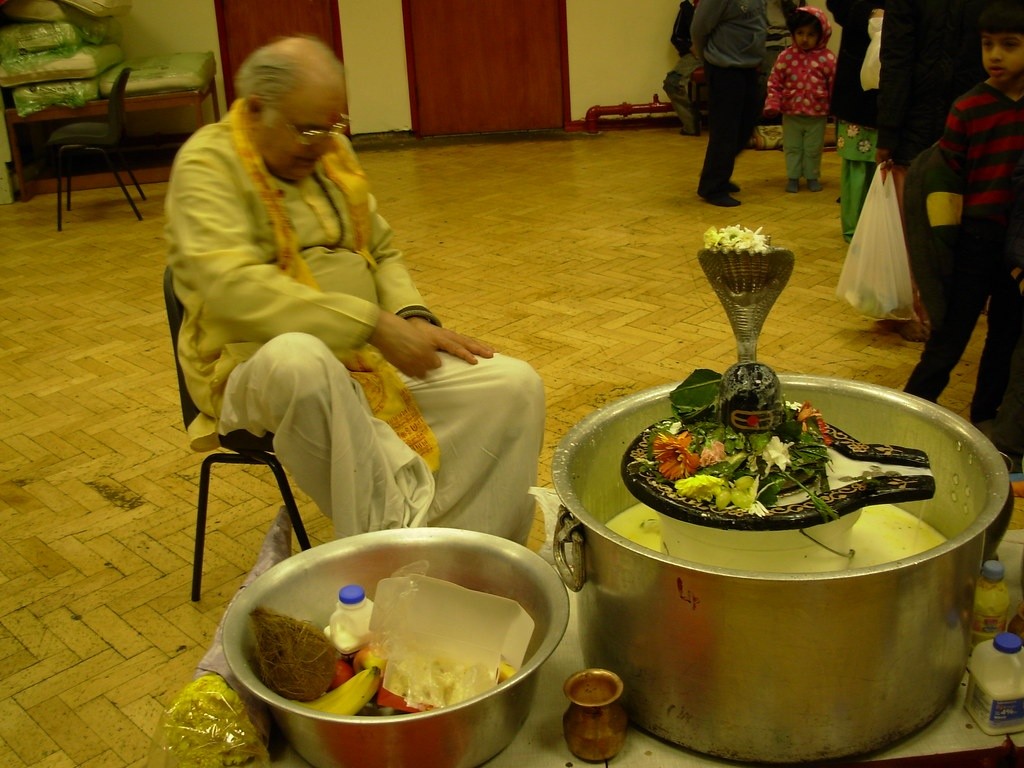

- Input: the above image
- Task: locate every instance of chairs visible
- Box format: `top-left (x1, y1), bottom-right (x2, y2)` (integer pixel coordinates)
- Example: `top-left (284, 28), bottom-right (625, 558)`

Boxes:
top-left (161, 262), bottom-right (311, 600)
top-left (45, 66), bottom-right (146, 232)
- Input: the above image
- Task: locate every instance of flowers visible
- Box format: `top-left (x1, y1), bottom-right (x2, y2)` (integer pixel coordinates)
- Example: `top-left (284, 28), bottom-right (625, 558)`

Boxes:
top-left (627, 368), bottom-right (843, 524)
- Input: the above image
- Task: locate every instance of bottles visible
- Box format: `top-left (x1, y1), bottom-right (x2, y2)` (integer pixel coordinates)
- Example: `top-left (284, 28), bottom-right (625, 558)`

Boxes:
top-left (968, 561), bottom-right (1010, 656)
top-left (964, 634), bottom-right (1024, 735)
top-left (323, 585), bottom-right (377, 665)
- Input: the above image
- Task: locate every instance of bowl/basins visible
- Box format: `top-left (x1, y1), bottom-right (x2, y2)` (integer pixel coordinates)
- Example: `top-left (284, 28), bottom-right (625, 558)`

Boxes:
top-left (224, 526), bottom-right (572, 767)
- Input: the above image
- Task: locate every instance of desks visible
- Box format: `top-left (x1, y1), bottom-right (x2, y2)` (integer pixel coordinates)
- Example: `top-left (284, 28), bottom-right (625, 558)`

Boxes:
top-left (482, 529), bottom-right (1024, 768)
top-left (5, 78), bottom-right (222, 201)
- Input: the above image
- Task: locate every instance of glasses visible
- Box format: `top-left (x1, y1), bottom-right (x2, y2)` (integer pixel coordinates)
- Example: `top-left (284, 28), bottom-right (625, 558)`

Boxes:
top-left (266, 103), bottom-right (350, 145)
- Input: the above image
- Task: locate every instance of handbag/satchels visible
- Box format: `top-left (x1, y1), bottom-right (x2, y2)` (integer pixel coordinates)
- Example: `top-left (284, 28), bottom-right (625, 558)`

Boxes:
top-left (837, 162), bottom-right (914, 321)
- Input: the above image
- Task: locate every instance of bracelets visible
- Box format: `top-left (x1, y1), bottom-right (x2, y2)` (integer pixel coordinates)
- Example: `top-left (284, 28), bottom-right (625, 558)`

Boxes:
top-left (399, 307), bottom-right (442, 328)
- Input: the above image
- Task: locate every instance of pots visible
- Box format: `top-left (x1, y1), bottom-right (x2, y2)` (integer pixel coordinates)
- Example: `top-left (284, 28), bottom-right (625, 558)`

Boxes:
top-left (552, 369), bottom-right (1015, 762)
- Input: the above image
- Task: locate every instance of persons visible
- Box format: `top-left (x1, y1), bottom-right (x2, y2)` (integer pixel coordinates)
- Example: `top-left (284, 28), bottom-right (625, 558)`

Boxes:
top-left (164, 35), bottom-right (545, 548)
top-left (827, 0), bottom-right (1024, 474)
top-left (662, 0), bottom-right (700, 137)
top-left (763, 5), bottom-right (838, 193)
top-left (688, 0), bottom-right (768, 207)
